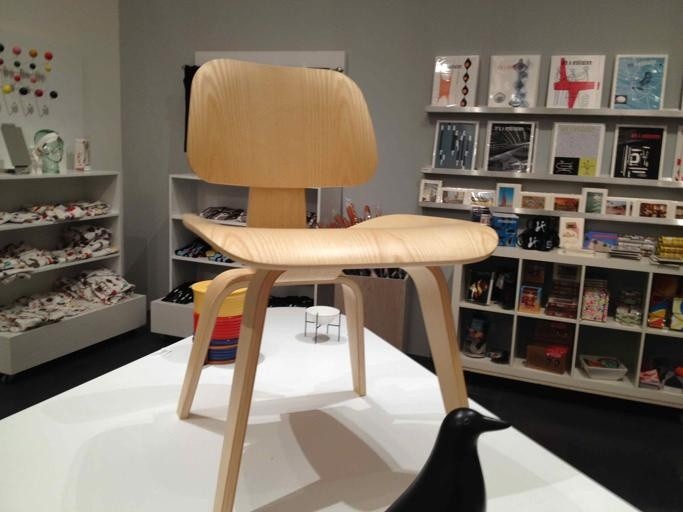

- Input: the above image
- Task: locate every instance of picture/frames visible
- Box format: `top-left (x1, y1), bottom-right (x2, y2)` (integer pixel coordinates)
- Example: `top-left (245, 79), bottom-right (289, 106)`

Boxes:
top-left (416, 118), bottom-right (683, 224)
top-left (608, 53), bottom-right (670, 112)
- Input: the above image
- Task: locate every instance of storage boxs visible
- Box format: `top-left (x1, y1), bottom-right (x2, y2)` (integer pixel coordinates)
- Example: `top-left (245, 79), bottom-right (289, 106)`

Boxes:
top-left (525, 340), bottom-right (569, 375)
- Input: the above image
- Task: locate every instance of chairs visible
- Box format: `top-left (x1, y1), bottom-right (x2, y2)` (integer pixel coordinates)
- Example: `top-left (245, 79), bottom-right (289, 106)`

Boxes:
top-left (176, 55), bottom-right (499, 512)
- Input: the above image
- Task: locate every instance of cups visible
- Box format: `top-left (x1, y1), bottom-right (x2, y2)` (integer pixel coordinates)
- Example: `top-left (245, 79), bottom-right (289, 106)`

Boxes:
top-left (72, 137), bottom-right (89, 170)
top-left (190, 279), bottom-right (249, 365)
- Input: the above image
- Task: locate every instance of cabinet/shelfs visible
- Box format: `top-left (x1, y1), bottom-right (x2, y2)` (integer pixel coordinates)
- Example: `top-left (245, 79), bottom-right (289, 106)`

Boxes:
top-left (416, 98), bottom-right (683, 229)
top-left (146, 172), bottom-right (346, 341)
top-left (450, 242), bottom-right (681, 413)
top-left (1, 166), bottom-right (146, 377)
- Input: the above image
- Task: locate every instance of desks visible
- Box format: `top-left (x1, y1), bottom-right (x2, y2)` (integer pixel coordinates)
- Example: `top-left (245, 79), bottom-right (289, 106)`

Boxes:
top-left (0, 302), bottom-right (643, 512)
